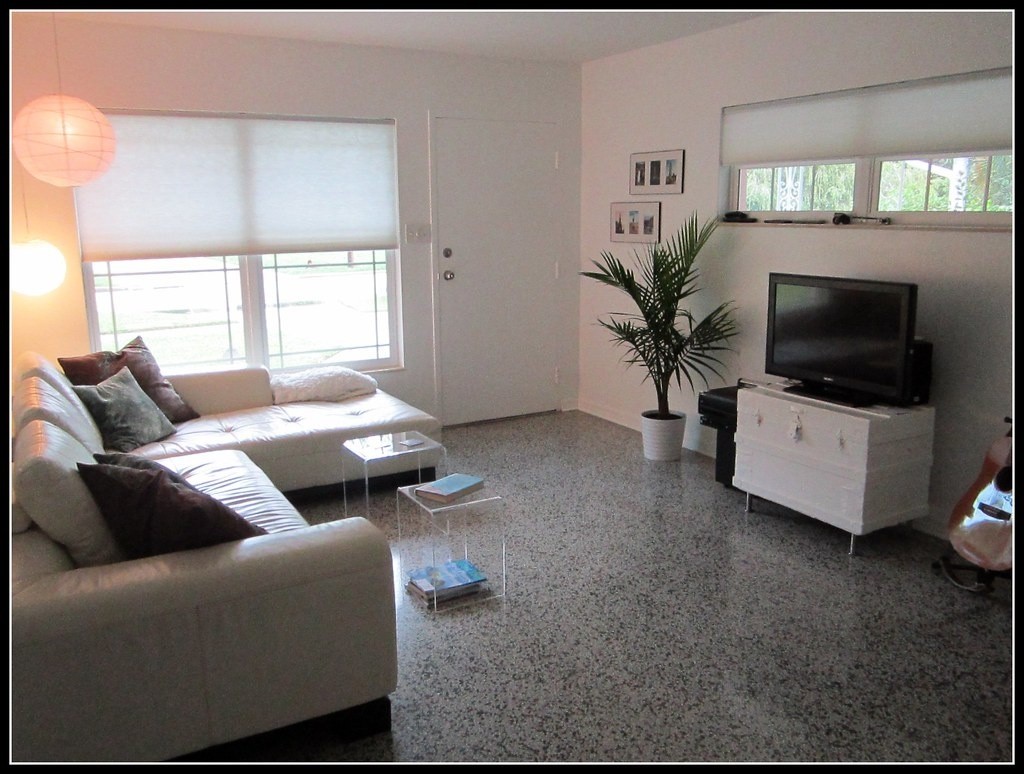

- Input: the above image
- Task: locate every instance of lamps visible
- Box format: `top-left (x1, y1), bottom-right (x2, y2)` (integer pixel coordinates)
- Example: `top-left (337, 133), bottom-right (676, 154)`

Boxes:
top-left (13, 12), bottom-right (116, 188)
top-left (11, 167), bottom-right (68, 297)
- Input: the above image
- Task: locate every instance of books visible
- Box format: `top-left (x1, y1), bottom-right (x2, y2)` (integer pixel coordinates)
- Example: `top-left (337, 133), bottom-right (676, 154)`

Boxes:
top-left (400, 558), bottom-right (487, 610)
top-left (415, 472), bottom-right (486, 506)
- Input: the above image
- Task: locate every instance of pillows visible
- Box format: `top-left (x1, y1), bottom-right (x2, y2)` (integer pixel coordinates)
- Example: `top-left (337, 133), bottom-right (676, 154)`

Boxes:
top-left (57, 336), bottom-right (201, 424)
top-left (75, 461), bottom-right (270, 559)
top-left (71, 365), bottom-right (177, 453)
top-left (92, 453), bottom-right (201, 492)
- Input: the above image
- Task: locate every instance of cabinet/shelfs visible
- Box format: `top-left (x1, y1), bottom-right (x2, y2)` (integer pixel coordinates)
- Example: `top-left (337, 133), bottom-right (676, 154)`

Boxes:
top-left (731, 382), bottom-right (936, 556)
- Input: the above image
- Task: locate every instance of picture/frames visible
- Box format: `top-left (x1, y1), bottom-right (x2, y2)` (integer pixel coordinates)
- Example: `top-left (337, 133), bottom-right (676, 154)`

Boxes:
top-left (609, 201), bottom-right (661, 244)
top-left (628, 149), bottom-right (685, 195)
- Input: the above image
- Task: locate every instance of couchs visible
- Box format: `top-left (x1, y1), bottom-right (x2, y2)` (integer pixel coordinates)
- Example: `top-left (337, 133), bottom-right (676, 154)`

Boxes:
top-left (12, 361), bottom-right (447, 762)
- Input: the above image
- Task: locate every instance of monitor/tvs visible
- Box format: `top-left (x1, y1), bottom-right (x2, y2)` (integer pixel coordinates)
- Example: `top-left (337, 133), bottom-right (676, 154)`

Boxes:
top-left (765, 271), bottom-right (917, 407)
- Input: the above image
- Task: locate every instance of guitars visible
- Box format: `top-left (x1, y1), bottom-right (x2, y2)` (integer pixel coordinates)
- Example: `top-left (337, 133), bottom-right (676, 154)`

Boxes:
top-left (949, 416), bottom-right (1014, 571)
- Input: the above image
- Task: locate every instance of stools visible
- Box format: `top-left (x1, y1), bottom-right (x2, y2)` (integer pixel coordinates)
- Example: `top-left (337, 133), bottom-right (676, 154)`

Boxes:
top-left (697, 384), bottom-right (740, 487)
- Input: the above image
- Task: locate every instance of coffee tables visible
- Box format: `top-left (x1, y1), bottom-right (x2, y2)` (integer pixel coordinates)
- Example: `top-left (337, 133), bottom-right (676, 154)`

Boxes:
top-left (395, 481), bottom-right (505, 614)
top-left (340, 430), bottom-right (448, 522)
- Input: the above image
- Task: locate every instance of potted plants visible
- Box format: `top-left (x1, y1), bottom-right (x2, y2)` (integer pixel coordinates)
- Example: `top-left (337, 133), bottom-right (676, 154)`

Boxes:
top-left (576, 207), bottom-right (742, 462)
